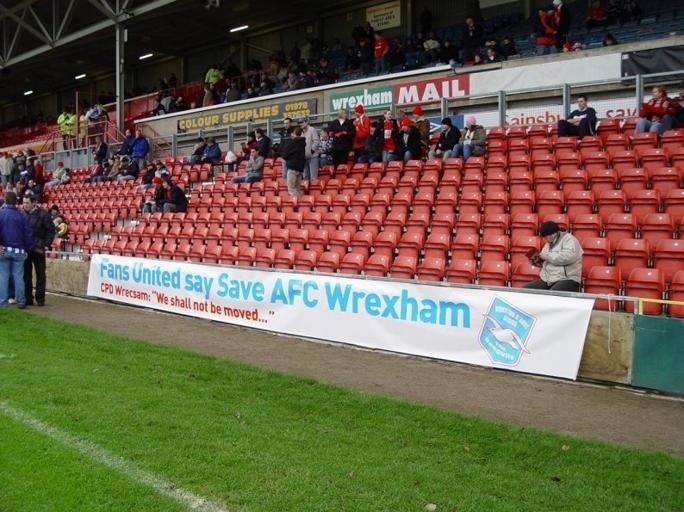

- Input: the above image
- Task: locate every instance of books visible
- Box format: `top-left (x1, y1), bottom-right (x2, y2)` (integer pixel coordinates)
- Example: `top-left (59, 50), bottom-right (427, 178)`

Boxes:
top-left (525, 246), bottom-right (542, 265)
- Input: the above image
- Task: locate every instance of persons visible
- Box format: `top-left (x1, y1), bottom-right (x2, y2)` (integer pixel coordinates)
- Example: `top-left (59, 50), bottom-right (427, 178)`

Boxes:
top-left (0, 144), bottom-right (72, 239)
top-left (602, 34), bottom-right (619, 49)
top-left (0, 192), bottom-right (27, 309)
top-left (634, 86), bottom-right (672, 134)
top-left (586, 0), bottom-right (609, 35)
top-left (522, 221), bottom-right (583, 292)
top-left (19, 194), bottom-right (55, 307)
top-left (670, 87), bottom-right (684, 128)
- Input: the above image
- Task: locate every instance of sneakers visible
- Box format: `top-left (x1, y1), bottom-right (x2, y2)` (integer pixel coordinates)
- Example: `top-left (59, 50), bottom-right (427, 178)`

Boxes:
top-left (1, 300), bottom-right (44, 308)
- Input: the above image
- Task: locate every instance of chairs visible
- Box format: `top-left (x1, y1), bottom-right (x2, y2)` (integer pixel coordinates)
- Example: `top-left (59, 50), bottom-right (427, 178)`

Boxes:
top-left (40, 115), bottom-right (684, 319)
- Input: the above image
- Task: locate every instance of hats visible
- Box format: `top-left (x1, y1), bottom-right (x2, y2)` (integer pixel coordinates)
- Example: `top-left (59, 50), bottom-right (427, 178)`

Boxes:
top-left (355, 105), bottom-right (363, 114)
top-left (413, 105), bottom-right (423, 115)
top-left (400, 118), bottom-right (412, 126)
top-left (151, 176), bottom-right (164, 184)
top-left (540, 221), bottom-right (558, 235)
